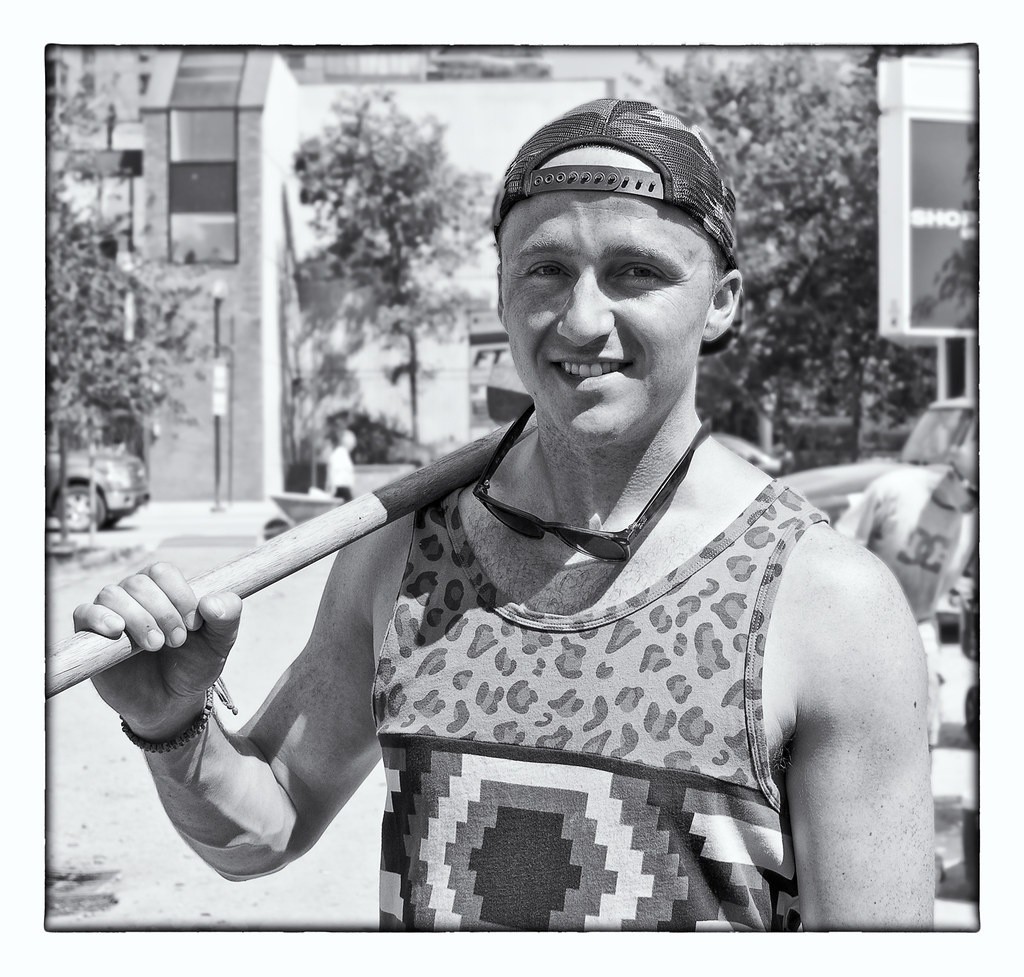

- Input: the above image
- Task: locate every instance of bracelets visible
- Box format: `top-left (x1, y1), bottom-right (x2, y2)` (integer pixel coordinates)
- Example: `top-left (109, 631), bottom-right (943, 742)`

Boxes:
top-left (118, 672), bottom-right (243, 755)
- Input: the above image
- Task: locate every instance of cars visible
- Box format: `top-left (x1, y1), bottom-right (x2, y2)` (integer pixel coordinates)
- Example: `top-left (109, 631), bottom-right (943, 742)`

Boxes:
top-left (772, 392), bottom-right (977, 529)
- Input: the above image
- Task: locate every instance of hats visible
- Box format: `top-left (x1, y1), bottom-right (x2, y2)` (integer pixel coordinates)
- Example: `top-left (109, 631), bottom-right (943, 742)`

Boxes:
top-left (492, 99), bottom-right (743, 357)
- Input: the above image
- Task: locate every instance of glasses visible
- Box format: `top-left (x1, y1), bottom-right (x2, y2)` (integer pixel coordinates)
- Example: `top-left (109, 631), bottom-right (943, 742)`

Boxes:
top-left (473, 403), bottom-right (711, 562)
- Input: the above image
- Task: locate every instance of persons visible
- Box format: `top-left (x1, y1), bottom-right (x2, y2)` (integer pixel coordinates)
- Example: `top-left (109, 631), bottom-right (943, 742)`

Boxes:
top-left (66, 94), bottom-right (941, 936)
top-left (833, 441), bottom-right (979, 657)
top-left (324, 429), bottom-right (357, 503)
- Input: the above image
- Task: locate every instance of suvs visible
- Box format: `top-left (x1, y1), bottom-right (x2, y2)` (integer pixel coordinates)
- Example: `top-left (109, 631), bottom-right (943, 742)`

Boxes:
top-left (44, 419), bottom-right (150, 536)
